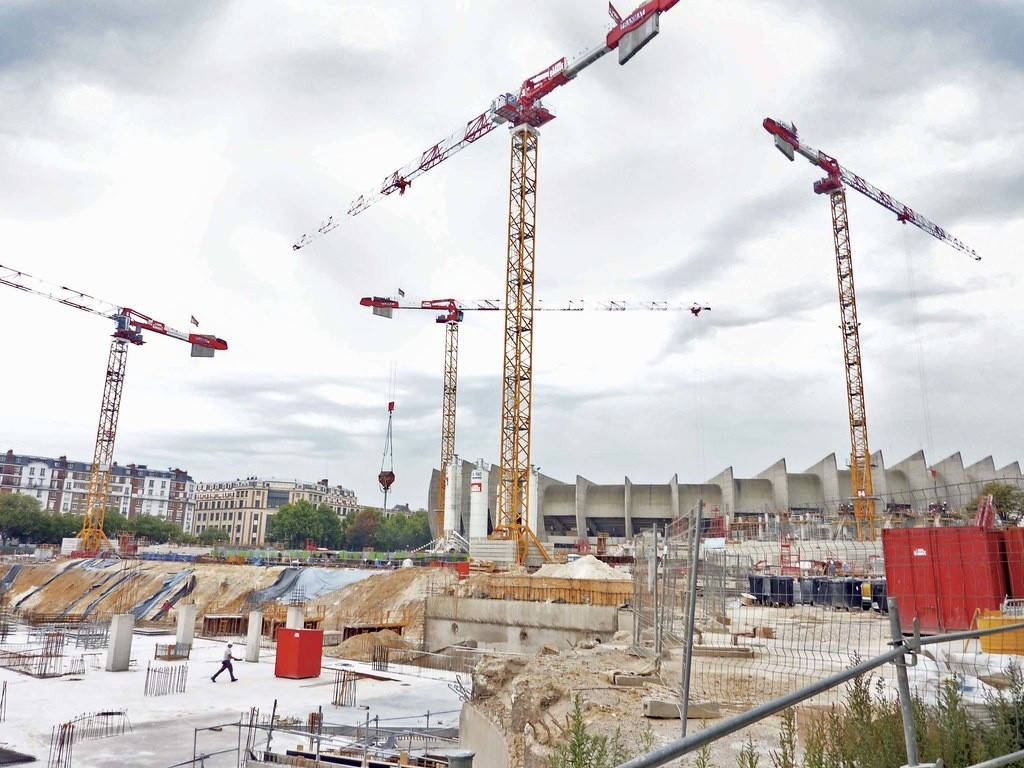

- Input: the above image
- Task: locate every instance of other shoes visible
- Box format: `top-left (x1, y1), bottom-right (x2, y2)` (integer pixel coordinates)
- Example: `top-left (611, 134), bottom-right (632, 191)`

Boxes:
top-left (211, 677), bottom-right (215, 682)
top-left (231, 678), bottom-right (238, 682)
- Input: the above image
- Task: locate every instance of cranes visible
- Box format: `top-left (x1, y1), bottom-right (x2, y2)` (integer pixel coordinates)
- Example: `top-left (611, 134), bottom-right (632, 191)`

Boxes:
top-left (0, 264), bottom-right (228, 558)
top-left (289, 0), bottom-right (679, 565)
top-left (359, 287), bottom-right (713, 553)
top-left (762, 118), bottom-right (982, 539)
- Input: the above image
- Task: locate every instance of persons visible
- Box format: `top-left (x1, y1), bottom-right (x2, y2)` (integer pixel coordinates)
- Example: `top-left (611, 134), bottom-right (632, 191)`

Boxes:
top-left (824, 563), bottom-right (828, 575)
top-left (211, 641), bottom-right (238, 682)
top-left (829, 561), bottom-right (835, 575)
top-left (812, 562), bottom-right (822, 572)
top-left (161, 600), bottom-right (174, 618)
top-left (156, 547), bottom-right (159, 553)
top-left (842, 562), bottom-right (849, 570)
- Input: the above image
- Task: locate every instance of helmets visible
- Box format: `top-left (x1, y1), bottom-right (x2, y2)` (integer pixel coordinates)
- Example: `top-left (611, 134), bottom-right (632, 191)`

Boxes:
top-left (228, 641), bottom-right (233, 644)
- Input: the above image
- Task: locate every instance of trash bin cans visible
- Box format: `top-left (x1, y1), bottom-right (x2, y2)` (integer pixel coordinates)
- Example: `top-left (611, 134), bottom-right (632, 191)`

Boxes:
top-left (798, 576), bottom-right (887, 609)
top-left (748, 574), bottom-right (794, 607)
